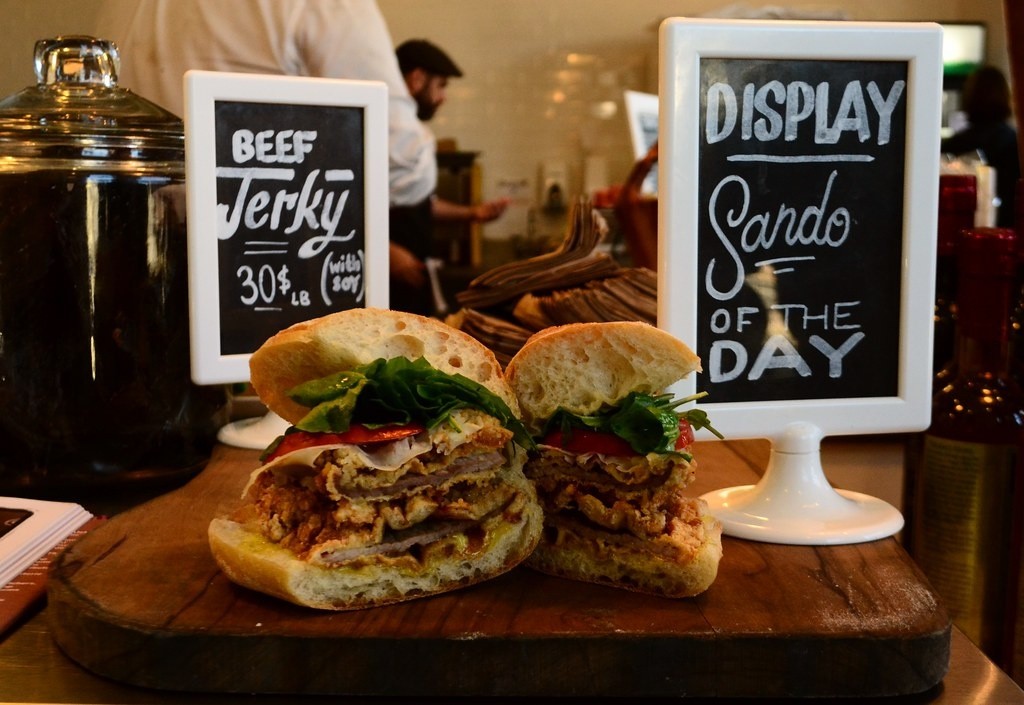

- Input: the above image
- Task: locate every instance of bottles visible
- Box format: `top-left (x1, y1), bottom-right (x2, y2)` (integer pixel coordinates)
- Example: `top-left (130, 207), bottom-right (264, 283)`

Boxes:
top-left (900, 226), bottom-right (1023, 673)
top-left (0, 33), bottom-right (218, 513)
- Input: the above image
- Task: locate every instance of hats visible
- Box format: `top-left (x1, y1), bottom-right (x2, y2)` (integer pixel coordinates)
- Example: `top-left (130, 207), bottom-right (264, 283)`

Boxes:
top-left (394, 40), bottom-right (462, 78)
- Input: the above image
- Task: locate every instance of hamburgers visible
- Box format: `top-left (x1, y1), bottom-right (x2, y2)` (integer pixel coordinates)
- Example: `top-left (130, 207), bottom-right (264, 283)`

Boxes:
top-left (502, 321), bottom-right (726, 598)
top-left (208, 309), bottom-right (546, 610)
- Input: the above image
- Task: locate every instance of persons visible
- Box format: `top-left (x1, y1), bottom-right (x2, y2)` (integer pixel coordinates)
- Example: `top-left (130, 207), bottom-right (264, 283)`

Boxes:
top-left (115, 0), bottom-right (440, 207)
top-left (941, 69), bottom-right (1020, 227)
top-left (389, 40), bottom-right (508, 311)
